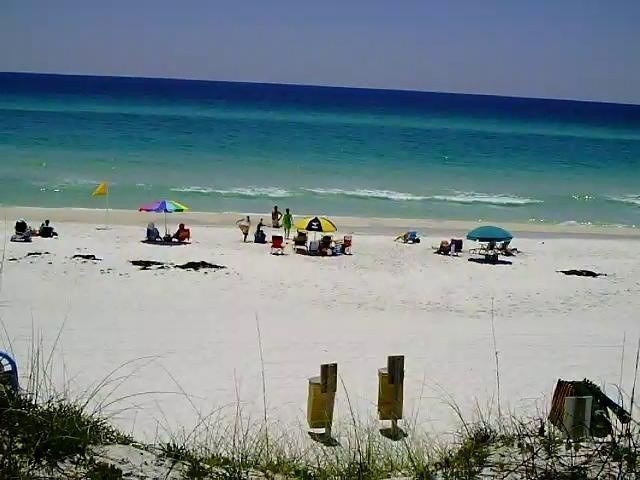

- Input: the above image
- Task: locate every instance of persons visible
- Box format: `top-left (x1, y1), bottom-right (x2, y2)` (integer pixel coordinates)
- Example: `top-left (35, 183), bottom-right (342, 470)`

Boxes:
top-left (147, 222), bottom-right (164, 241)
top-left (271, 206), bottom-right (283, 228)
top-left (172, 224), bottom-right (185, 239)
top-left (256, 217), bottom-right (271, 233)
top-left (139, 200), bottom-right (192, 235)
top-left (283, 208), bottom-right (294, 238)
top-left (235, 215), bottom-right (252, 242)
top-left (40, 219), bottom-right (50, 232)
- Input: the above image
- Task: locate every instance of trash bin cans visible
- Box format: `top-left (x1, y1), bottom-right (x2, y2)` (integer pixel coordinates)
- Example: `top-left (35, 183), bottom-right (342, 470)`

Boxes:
top-left (307, 363), bottom-right (337, 441)
top-left (378, 356), bottom-right (404, 438)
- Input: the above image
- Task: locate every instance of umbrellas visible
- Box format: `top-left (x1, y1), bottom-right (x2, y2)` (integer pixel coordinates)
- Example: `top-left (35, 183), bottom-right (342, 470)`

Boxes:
top-left (295, 216), bottom-right (338, 241)
top-left (467, 225), bottom-right (514, 243)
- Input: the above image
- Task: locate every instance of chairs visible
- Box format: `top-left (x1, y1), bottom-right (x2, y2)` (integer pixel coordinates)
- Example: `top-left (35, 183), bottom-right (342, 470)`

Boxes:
top-left (270, 235), bottom-right (284, 255)
top-left (469, 240), bottom-right (510, 255)
top-left (437, 239), bottom-right (463, 257)
top-left (292, 231), bottom-right (352, 257)
top-left (15, 221), bottom-right (30, 239)
top-left (37, 227), bottom-right (53, 237)
top-left (394, 230), bottom-right (418, 243)
top-left (146, 224), bottom-right (190, 242)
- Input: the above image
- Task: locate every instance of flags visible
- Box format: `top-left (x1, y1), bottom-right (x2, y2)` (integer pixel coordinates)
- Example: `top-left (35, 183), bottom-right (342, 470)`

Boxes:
top-left (92, 182), bottom-right (108, 196)
